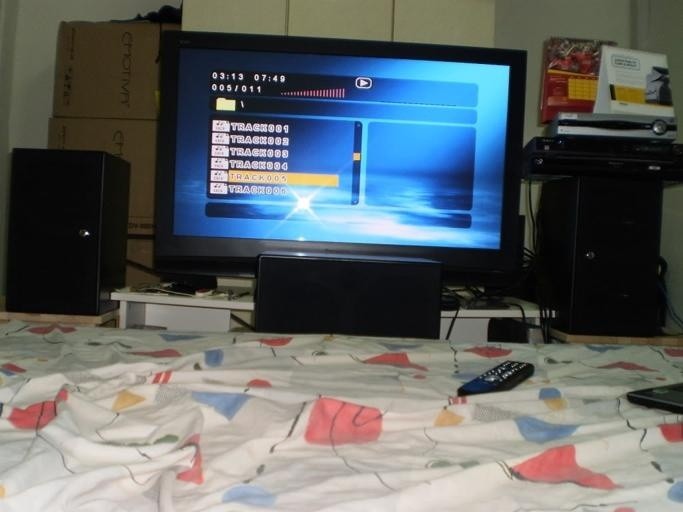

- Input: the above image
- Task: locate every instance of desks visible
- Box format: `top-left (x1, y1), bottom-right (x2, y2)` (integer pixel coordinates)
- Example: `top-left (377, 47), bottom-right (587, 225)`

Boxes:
top-left (110, 282), bottom-right (560, 342)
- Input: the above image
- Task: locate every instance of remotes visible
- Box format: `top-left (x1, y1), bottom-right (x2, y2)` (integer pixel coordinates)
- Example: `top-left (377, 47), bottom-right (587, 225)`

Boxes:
top-left (458, 361), bottom-right (534, 398)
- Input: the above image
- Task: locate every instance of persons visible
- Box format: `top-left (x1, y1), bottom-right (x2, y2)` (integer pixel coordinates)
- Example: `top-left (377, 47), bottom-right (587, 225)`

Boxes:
top-left (565, 42), bottom-right (600, 73)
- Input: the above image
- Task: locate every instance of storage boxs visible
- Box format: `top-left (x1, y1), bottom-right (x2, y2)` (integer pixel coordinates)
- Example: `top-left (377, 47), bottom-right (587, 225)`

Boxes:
top-left (47, 20), bottom-right (179, 292)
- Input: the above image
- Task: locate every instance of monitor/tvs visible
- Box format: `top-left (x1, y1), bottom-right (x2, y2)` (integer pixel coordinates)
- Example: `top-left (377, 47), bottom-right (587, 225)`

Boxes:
top-left (152, 29), bottom-right (527, 287)
